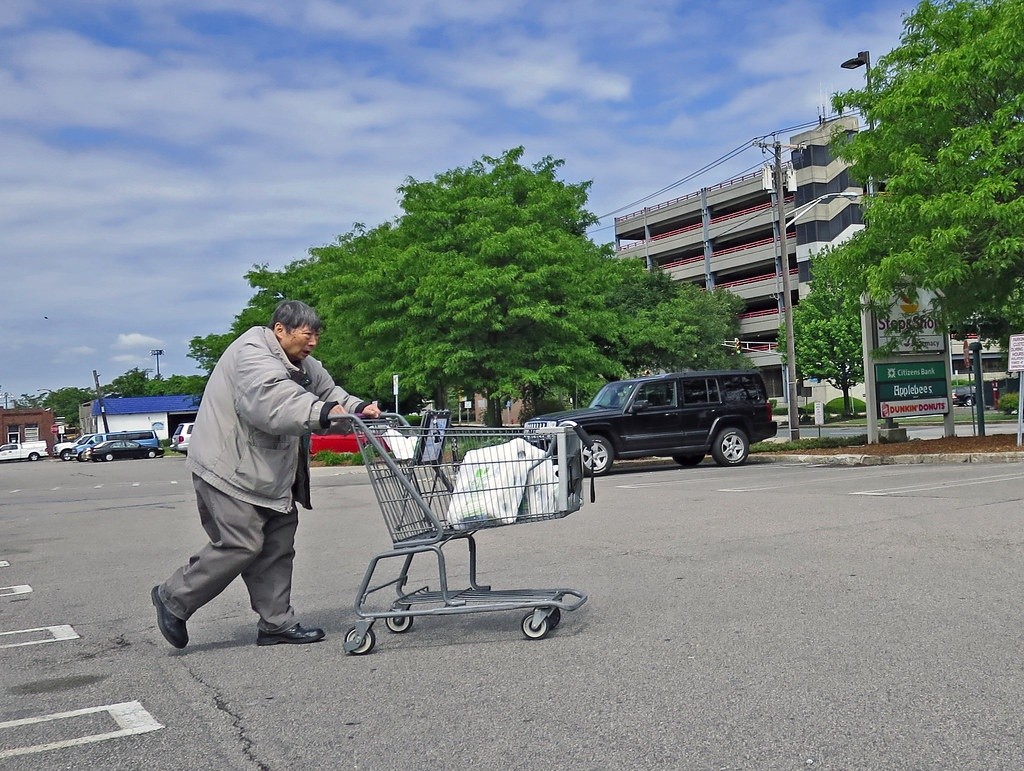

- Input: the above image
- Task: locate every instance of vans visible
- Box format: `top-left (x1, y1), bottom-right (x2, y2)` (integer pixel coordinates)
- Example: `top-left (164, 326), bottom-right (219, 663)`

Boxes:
top-left (72, 430), bottom-right (159, 462)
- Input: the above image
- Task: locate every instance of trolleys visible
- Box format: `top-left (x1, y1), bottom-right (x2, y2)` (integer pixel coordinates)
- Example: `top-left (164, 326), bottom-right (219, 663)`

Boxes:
top-left (327, 412), bottom-right (587, 655)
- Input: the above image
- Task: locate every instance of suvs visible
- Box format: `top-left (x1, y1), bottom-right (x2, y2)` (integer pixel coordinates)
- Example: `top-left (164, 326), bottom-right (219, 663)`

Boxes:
top-left (524, 370), bottom-right (777, 477)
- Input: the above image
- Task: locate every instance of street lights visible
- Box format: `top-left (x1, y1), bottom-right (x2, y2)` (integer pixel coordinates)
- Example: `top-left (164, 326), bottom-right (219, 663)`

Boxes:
top-left (150, 349), bottom-right (165, 375)
top-left (778, 192), bottom-right (862, 443)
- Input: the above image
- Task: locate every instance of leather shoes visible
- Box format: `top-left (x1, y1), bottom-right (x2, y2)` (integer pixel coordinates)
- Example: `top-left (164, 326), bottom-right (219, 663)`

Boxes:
top-left (258, 624), bottom-right (324, 644)
top-left (151, 586), bottom-right (189, 648)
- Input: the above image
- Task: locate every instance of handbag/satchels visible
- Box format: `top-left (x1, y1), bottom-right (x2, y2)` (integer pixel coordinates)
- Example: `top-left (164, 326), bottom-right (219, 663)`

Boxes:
top-left (447, 438), bottom-right (560, 529)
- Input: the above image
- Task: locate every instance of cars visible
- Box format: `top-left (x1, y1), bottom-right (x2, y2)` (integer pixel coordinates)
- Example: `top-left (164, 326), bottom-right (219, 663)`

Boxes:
top-left (170, 423), bottom-right (196, 455)
top-left (82, 441), bottom-right (111, 462)
top-left (952, 385), bottom-right (975, 407)
top-left (52, 434), bottom-right (93, 461)
top-left (309, 414), bottom-right (390, 457)
top-left (90, 440), bottom-right (165, 462)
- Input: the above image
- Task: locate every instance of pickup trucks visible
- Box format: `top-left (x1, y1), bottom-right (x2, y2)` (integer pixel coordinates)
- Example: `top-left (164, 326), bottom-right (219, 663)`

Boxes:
top-left (0, 440), bottom-right (50, 461)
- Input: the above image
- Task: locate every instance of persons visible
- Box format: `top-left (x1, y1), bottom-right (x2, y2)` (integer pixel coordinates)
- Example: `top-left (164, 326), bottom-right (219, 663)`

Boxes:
top-left (151, 299), bottom-right (382, 649)
